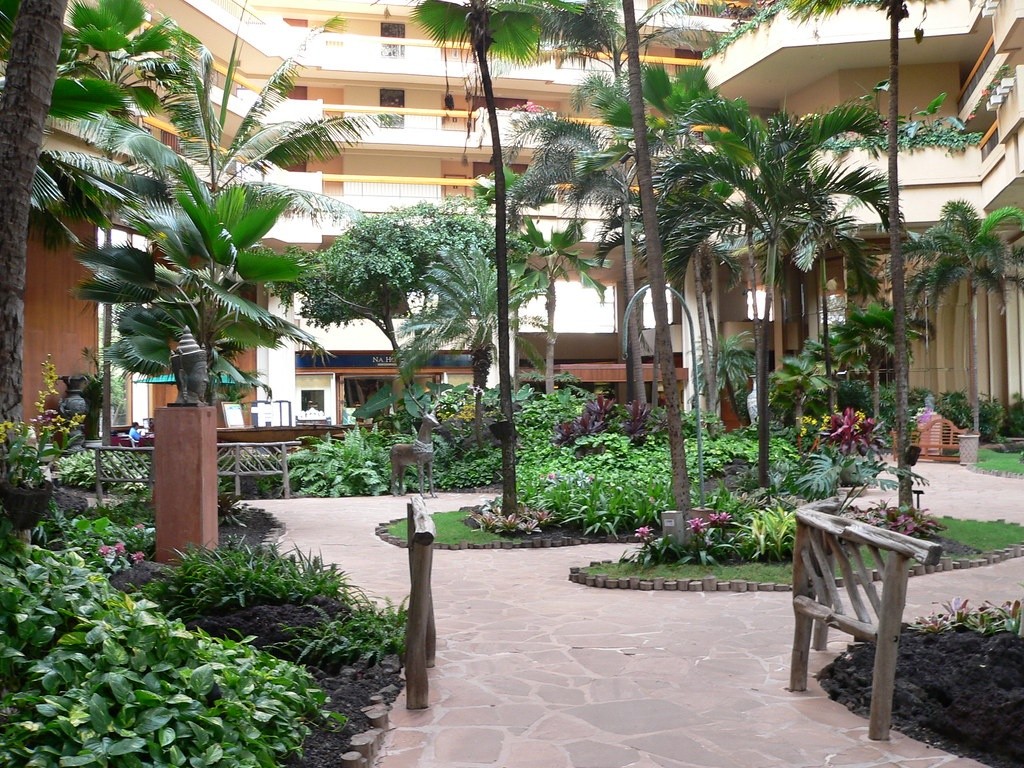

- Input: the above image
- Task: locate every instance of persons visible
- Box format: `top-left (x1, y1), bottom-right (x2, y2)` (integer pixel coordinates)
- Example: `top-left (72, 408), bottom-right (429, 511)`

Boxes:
top-left (170, 324), bottom-right (210, 403)
top-left (148, 418), bottom-right (155, 433)
top-left (130, 422), bottom-right (144, 447)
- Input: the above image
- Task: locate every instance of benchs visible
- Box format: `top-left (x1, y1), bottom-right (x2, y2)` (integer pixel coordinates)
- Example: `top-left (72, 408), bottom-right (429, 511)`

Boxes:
top-left (890, 419), bottom-right (981, 463)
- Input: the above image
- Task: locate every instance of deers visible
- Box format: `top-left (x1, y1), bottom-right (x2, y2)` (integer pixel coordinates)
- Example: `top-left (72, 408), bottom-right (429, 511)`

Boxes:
top-left (388, 384), bottom-right (444, 501)
